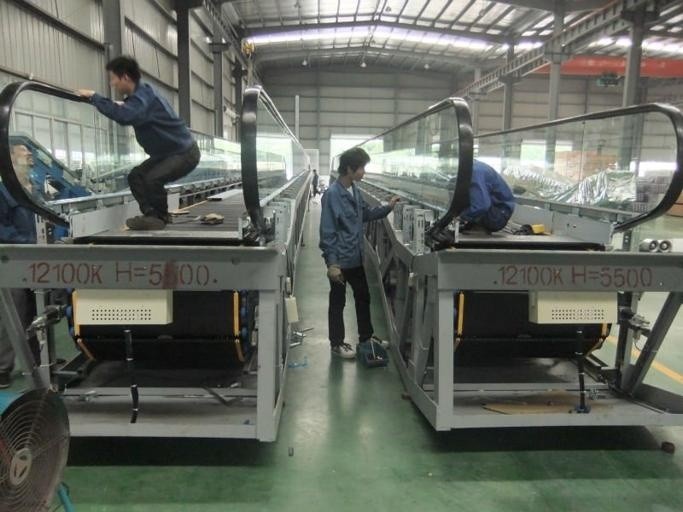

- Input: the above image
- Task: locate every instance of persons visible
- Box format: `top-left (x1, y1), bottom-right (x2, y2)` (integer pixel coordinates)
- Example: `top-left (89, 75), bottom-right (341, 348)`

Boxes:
top-left (0, 144), bottom-right (37, 243)
top-left (459, 159), bottom-right (515, 235)
top-left (76, 56), bottom-right (200, 230)
top-left (312, 169), bottom-right (320, 196)
top-left (319, 147), bottom-right (401, 359)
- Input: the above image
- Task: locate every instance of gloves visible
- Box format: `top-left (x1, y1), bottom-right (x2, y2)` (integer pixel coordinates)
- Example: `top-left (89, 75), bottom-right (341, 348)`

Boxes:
top-left (327, 265), bottom-right (345, 286)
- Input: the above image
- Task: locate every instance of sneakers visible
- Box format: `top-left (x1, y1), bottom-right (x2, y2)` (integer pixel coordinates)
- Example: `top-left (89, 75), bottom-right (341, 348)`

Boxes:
top-left (125, 215), bottom-right (166, 230)
top-left (0, 373), bottom-right (9, 389)
top-left (459, 222), bottom-right (489, 235)
top-left (359, 334), bottom-right (389, 349)
top-left (331, 344), bottom-right (356, 358)
